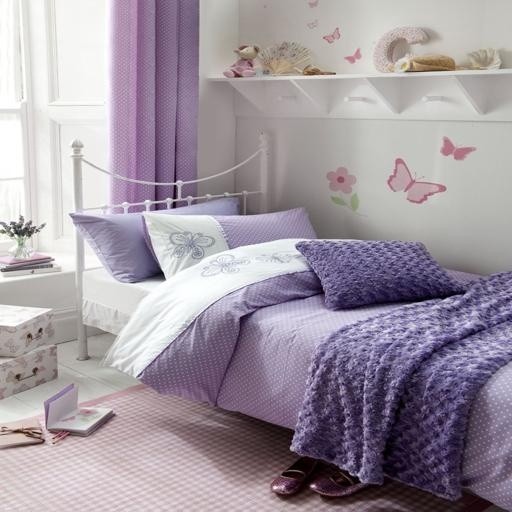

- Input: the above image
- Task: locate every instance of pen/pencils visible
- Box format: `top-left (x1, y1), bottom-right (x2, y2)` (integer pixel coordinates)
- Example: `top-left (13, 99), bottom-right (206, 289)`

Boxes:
top-left (52, 431), bottom-right (70, 444)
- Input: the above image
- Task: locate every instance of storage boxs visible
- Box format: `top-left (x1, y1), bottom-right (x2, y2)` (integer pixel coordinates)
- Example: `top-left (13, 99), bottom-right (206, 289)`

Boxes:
top-left (1, 305), bottom-right (59, 400)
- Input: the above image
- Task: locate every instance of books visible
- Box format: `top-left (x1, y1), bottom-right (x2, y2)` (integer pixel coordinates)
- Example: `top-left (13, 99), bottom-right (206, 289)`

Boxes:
top-left (43, 383), bottom-right (115, 438)
top-left (0, 416), bottom-right (45, 450)
top-left (0, 254), bottom-right (62, 277)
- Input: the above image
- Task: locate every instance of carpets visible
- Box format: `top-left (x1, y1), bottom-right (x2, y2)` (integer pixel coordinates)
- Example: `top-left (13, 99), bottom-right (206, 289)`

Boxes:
top-left (1, 386), bottom-right (508, 512)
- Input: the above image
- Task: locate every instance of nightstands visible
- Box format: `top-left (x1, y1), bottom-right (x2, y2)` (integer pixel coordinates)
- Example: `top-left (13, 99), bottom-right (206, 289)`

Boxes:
top-left (0, 262), bottom-right (77, 346)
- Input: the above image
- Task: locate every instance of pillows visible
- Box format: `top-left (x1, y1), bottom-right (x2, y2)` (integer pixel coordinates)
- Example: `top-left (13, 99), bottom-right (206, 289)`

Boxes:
top-left (295, 239), bottom-right (467, 310)
top-left (70, 197), bottom-right (238, 283)
top-left (141, 207), bottom-right (318, 281)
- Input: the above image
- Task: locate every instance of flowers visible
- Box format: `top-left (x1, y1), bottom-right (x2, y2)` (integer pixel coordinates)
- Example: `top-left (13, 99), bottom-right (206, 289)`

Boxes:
top-left (0, 215), bottom-right (47, 258)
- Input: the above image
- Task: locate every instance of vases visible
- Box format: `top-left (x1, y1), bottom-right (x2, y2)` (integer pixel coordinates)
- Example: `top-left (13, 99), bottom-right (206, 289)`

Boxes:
top-left (8, 236), bottom-right (35, 259)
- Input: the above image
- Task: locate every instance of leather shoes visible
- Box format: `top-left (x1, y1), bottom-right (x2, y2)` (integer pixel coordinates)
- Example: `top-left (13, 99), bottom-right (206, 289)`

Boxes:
top-left (269, 457), bottom-right (317, 497)
top-left (309, 470), bottom-right (368, 497)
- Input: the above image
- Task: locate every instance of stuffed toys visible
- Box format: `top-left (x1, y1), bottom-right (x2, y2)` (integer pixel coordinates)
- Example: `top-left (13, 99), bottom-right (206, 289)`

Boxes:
top-left (222, 42), bottom-right (259, 79)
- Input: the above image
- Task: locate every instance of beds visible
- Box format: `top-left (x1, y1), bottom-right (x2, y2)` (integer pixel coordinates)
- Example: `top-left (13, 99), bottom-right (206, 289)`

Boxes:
top-left (70, 135), bottom-right (512, 512)
